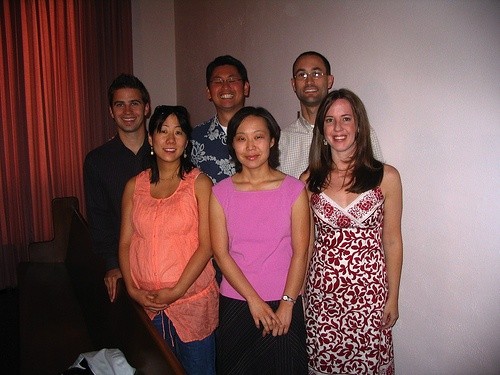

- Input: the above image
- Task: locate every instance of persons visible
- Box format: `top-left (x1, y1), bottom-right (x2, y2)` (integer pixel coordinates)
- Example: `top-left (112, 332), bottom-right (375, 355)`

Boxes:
top-left (118, 104), bottom-right (218, 375)
top-left (84, 72), bottom-right (156, 304)
top-left (272, 50), bottom-right (382, 179)
top-left (108, 53), bottom-right (249, 187)
top-left (299, 89), bottom-right (403, 375)
top-left (209, 105), bottom-right (310, 375)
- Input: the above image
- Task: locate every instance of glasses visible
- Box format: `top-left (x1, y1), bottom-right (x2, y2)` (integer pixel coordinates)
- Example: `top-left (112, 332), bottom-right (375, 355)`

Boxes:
top-left (294, 70), bottom-right (328, 81)
top-left (154, 106), bottom-right (188, 117)
top-left (208, 77), bottom-right (243, 87)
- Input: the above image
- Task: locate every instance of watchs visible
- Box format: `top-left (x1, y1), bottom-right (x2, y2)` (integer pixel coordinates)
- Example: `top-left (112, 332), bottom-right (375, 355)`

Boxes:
top-left (281, 295), bottom-right (296, 304)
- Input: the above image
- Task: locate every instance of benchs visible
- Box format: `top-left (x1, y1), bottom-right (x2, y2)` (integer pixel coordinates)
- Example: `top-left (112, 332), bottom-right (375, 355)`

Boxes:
top-left (18, 197), bottom-right (185, 375)
